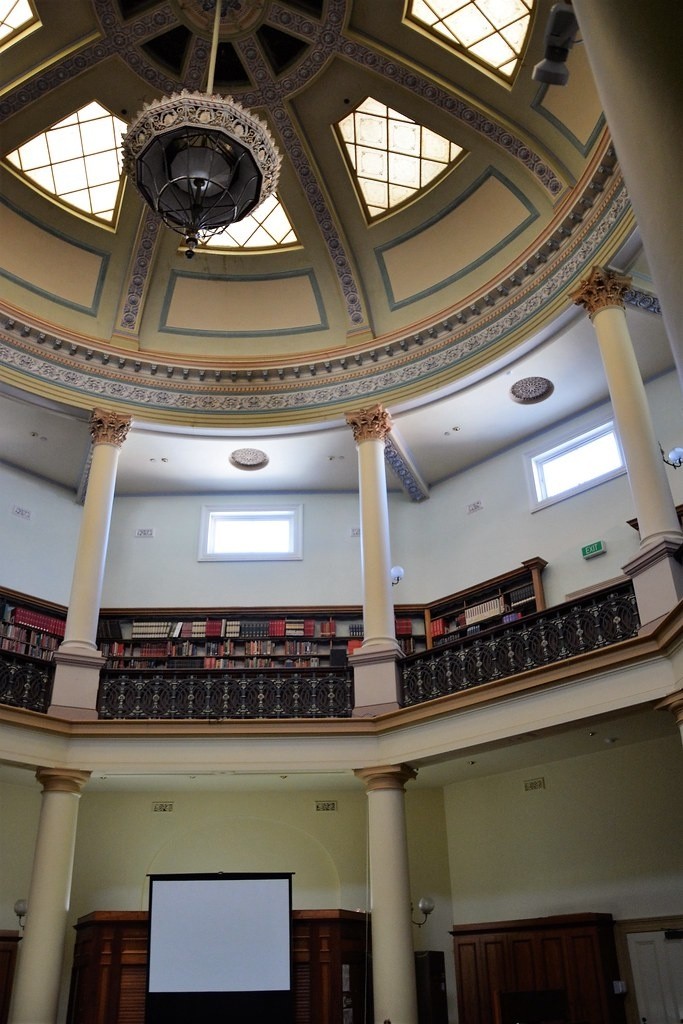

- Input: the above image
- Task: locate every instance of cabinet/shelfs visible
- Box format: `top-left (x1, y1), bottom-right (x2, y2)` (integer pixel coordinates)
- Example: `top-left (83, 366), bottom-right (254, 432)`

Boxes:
top-left (0, 556), bottom-right (549, 668)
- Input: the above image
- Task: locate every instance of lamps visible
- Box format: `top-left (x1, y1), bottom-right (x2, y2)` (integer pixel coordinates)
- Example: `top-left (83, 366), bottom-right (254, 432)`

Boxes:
top-left (409, 896), bottom-right (435, 928)
top-left (122, 0), bottom-right (284, 261)
top-left (13, 899), bottom-right (29, 932)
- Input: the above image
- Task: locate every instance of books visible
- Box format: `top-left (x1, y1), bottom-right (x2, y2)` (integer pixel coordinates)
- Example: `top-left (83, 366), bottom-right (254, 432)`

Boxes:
top-left (394, 585), bottom-right (535, 655)
top-left (98, 619), bottom-right (363, 668)
top-left (1, 607), bottom-right (67, 661)
